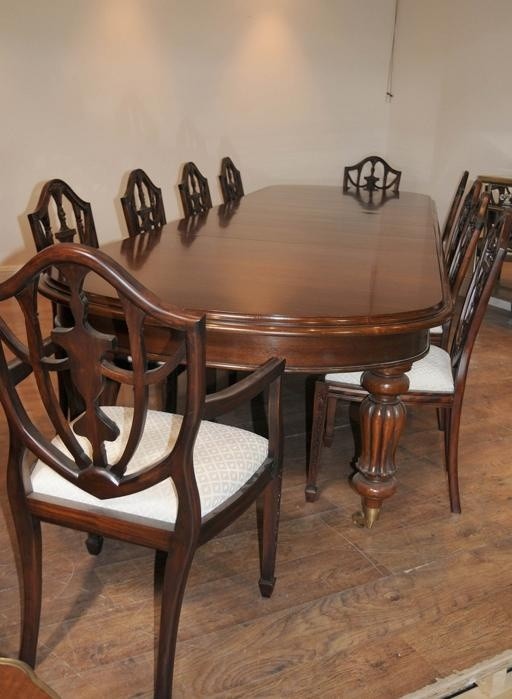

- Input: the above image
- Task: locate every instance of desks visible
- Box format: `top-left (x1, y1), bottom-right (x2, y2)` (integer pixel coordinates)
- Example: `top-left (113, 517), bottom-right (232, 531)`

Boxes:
top-left (35, 182), bottom-right (455, 532)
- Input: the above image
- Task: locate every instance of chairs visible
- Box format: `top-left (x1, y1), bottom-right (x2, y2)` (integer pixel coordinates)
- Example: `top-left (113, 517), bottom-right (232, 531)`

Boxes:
top-left (217, 154), bottom-right (246, 204)
top-left (177, 160), bottom-right (240, 405)
top-left (342, 155), bottom-right (403, 194)
top-left (25, 178), bottom-right (107, 557)
top-left (302, 168), bottom-right (512, 520)
top-left (120, 167), bottom-right (218, 413)
top-left (2, 241), bottom-right (284, 699)
top-left (3, 655), bottom-right (66, 699)
